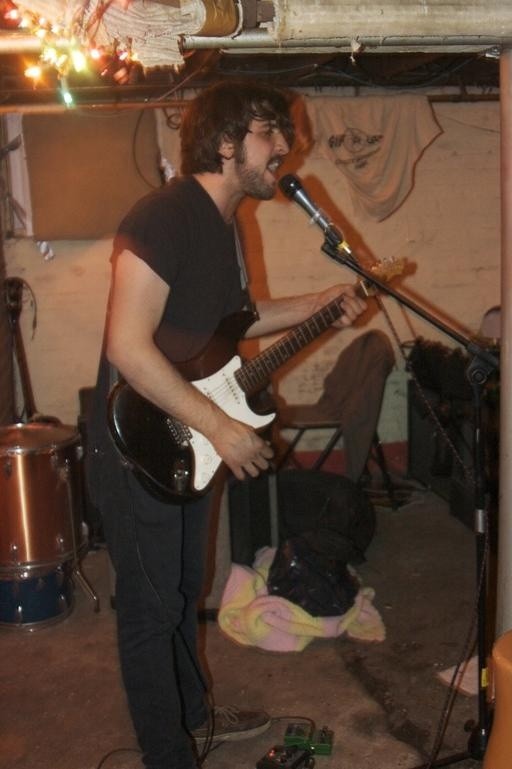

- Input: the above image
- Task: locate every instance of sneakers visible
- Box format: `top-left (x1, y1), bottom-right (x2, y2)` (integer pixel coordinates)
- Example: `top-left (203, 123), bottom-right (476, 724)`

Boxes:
top-left (189, 702), bottom-right (272, 744)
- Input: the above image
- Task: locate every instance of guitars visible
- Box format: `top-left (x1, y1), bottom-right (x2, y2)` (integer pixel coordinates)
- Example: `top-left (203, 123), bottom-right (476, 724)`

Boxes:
top-left (108, 255), bottom-right (411, 501)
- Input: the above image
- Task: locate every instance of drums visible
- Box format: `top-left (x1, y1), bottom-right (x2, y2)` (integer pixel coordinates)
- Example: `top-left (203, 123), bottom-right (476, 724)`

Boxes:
top-left (0, 419), bottom-right (87, 580)
top-left (1, 568), bottom-right (76, 631)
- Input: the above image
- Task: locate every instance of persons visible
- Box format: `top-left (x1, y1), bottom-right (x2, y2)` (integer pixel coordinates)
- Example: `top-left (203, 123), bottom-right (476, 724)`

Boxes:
top-left (83, 79), bottom-right (365, 769)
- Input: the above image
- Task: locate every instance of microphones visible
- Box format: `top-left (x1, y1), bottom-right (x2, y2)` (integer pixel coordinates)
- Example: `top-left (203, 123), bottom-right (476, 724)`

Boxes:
top-left (279, 172), bottom-right (343, 247)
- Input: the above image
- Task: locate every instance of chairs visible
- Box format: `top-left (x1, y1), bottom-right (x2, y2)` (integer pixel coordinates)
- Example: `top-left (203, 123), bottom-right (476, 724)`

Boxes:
top-left (274, 327), bottom-right (402, 513)
